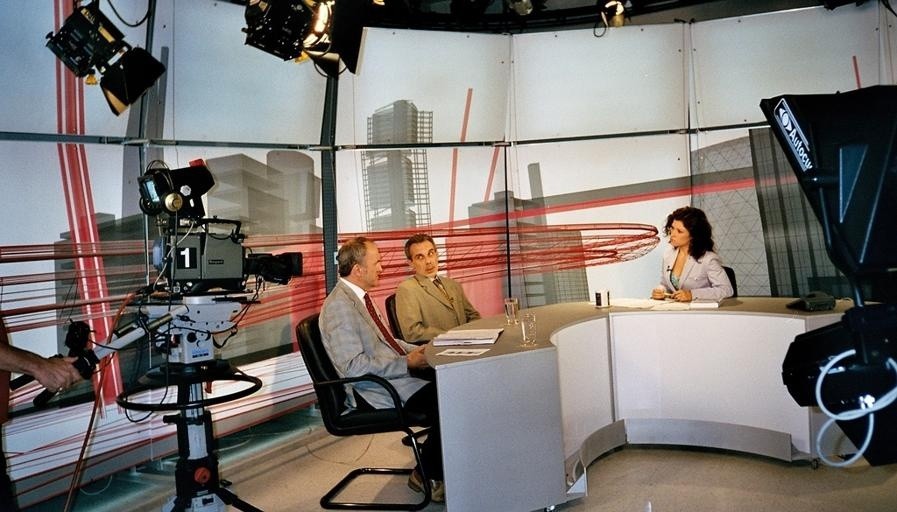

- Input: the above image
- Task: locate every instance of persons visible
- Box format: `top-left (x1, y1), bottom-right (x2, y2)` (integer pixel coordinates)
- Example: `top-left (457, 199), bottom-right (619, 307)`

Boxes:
top-left (652, 206), bottom-right (734, 303)
top-left (395, 233), bottom-right (482, 344)
top-left (0, 314), bottom-right (83, 512)
top-left (317, 237), bottom-right (445, 505)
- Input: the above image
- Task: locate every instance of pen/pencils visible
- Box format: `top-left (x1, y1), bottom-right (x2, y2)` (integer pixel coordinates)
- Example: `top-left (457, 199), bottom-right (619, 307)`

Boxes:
top-left (664, 292), bottom-right (672, 296)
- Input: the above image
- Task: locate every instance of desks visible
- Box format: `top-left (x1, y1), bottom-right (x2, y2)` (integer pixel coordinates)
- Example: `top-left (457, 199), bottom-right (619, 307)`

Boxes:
top-left (603, 292), bottom-right (872, 481)
top-left (405, 300), bottom-right (628, 511)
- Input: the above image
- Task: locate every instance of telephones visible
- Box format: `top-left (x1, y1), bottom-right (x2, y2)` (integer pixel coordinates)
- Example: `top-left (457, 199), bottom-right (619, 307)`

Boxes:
top-left (786, 291), bottom-right (835, 312)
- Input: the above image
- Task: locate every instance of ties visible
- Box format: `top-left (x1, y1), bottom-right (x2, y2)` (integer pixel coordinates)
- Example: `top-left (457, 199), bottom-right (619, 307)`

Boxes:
top-left (363, 293), bottom-right (406, 356)
top-left (433, 278), bottom-right (450, 299)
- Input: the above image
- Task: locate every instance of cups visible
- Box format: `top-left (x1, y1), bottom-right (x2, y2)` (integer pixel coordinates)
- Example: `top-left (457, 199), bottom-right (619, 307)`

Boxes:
top-left (594, 289), bottom-right (609, 307)
top-left (519, 311), bottom-right (538, 344)
top-left (503, 296), bottom-right (520, 326)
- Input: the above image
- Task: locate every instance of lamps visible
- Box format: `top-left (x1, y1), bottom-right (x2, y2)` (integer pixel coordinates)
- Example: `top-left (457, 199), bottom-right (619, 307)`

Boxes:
top-left (588, 1), bottom-right (631, 38)
top-left (240, 0), bottom-right (366, 83)
top-left (41, 1), bottom-right (172, 118)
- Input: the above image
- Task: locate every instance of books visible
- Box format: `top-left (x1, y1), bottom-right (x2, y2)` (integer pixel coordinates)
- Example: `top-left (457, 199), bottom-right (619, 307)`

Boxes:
top-left (432, 328), bottom-right (504, 347)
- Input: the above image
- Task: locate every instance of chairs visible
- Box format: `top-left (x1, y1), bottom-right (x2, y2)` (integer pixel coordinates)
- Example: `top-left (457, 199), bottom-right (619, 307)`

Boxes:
top-left (719, 264), bottom-right (741, 299)
top-left (381, 290), bottom-right (430, 346)
top-left (286, 307), bottom-right (437, 511)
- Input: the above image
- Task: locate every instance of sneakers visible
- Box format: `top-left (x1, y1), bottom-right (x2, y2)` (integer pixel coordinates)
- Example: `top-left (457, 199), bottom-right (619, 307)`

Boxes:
top-left (408, 469), bottom-right (446, 503)
